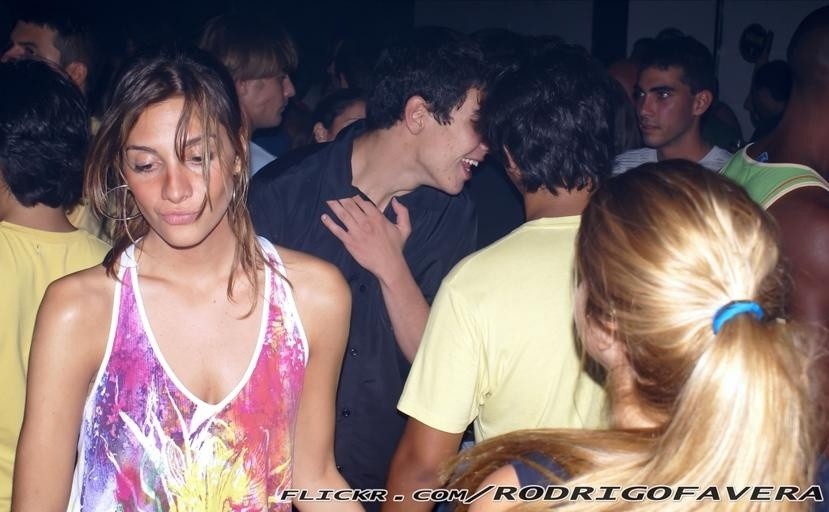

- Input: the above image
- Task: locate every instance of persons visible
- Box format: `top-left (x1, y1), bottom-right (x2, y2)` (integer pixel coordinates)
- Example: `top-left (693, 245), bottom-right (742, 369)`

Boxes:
top-left (1, 3), bottom-right (829, 511)
